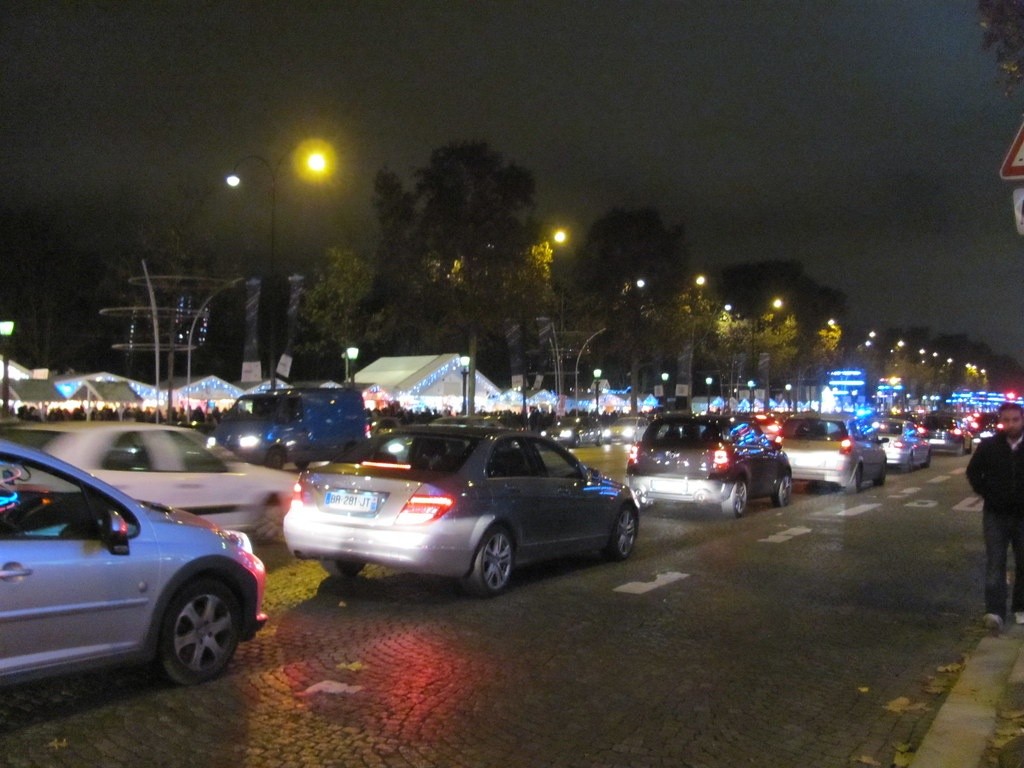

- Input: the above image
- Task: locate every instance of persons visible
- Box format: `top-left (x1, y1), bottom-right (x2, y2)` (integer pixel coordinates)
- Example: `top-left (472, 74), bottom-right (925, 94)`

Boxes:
top-left (965, 403), bottom-right (1023, 631)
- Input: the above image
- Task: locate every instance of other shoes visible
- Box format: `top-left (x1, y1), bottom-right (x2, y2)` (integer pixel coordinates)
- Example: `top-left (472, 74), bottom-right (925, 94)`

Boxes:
top-left (1013, 612), bottom-right (1024, 625)
top-left (982, 613), bottom-right (1004, 636)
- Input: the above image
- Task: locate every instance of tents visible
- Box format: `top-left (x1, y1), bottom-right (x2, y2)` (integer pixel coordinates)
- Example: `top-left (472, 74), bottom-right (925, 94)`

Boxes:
top-left (0, 353), bottom-right (33, 385)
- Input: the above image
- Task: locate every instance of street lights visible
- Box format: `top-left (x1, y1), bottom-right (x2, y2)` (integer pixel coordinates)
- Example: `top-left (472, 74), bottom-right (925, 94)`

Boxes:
top-left (593, 367), bottom-right (602, 412)
top-left (706, 376), bottom-right (712, 412)
top-left (725, 299), bottom-right (782, 416)
top-left (785, 383), bottom-right (792, 412)
top-left (661, 372), bottom-right (669, 412)
top-left (0, 315), bottom-right (15, 414)
top-left (460, 351), bottom-right (470, 415)
top-left (347, 342), bottom-right (359, 389)
top-left (226, 141), bottom-right (328, 391)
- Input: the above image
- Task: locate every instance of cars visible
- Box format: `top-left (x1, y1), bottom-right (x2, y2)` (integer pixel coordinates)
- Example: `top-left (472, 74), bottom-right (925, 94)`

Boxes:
top-left (0, 439), bottom-right (268, 687)
top-left (627, 412), bottom-right (792, 518)
top-left (285, 426), bottom-right (642, 599)
top-left (969, 412), bottom-right (1003, 440)
top-left (0, 420), bottom-right (299, 544)
top-left (866, 419), bottom-right (931, 472)
top-left (603, 418), bottom-right (650, 446)
top-left (774, 414), bottom-right (889, 494)
top-left (918, 415), bottom-right (973, 457)
top-left (541, 416), bottom-right (604, 448)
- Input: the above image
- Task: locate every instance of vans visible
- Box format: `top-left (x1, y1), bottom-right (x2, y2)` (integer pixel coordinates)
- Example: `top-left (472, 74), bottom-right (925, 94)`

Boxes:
top-left (208, 388), bottom-right (371, 471)
